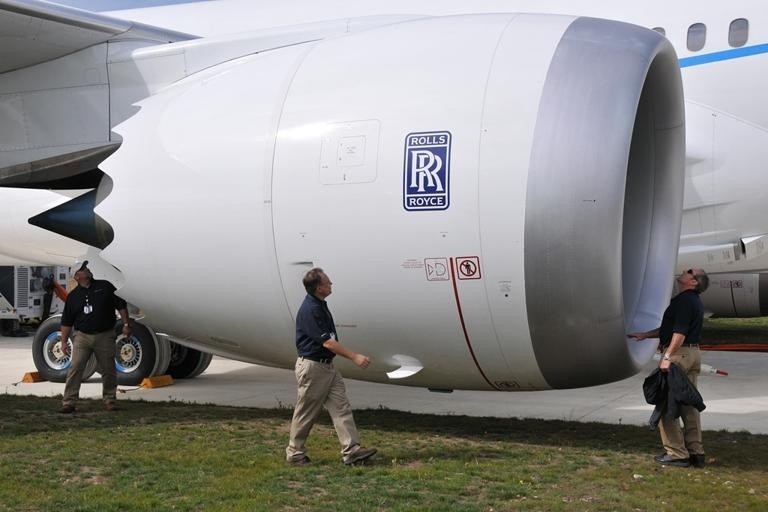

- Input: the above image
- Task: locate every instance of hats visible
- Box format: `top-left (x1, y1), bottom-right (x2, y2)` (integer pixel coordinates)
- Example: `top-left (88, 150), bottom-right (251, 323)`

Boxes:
top-left (69, 259), bottom-right (89, 279)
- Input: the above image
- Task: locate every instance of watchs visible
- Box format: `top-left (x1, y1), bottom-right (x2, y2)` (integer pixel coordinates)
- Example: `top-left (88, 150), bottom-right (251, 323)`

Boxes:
top-left (122, 324), bottom-right (130, 328)
top-left (663, 355), bottom-right (670, 360)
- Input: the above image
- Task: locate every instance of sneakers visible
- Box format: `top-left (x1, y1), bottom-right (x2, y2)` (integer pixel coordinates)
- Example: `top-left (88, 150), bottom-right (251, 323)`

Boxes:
top-left (104, 402), bottom-right (120, 410)
top-left (57, 404), bottom-right (75, 413)
top-left (654, 451), bottom-right (705, 468)
top-left (293, 456), bottom-right (315, 466)
top-left (343, 445), bottom-right (378, 466)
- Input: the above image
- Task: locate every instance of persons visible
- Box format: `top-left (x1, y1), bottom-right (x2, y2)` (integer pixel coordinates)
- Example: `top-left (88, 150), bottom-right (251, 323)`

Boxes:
top-left (626, 269), bottom-right (709, 466)
top-left (58, 260), bottom-right (132, 413)
top-left (287, 268), bottom-right (377, 467)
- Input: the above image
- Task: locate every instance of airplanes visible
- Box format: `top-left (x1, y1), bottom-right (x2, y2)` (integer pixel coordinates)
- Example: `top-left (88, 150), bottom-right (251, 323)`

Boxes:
top-left (0, 1), bottom-right (768, 392)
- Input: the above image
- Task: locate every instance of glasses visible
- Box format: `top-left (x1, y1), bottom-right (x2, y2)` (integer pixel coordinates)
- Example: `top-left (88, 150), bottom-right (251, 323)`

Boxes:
top-left (687, 268), bottom-right (697, 281)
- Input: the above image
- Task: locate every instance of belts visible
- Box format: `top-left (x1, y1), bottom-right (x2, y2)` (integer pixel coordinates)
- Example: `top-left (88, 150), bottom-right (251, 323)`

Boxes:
top-left (661, 343), bottom-right (698, 348)
top-left (299, 355), bottom-right (333, 364)
